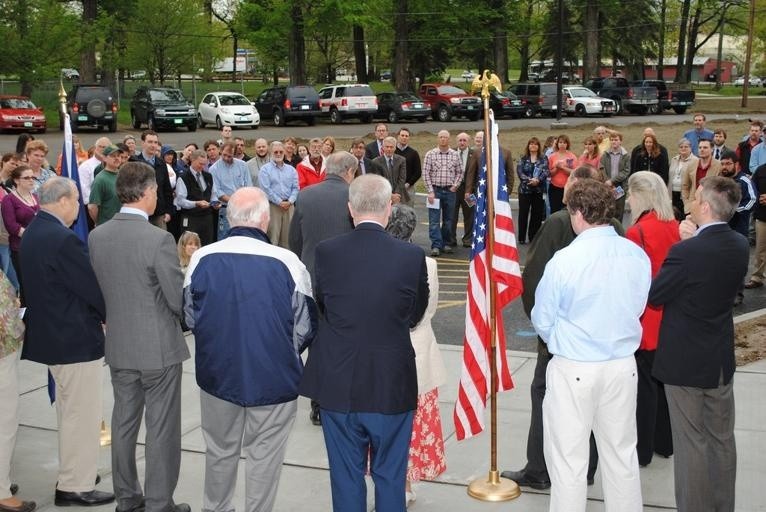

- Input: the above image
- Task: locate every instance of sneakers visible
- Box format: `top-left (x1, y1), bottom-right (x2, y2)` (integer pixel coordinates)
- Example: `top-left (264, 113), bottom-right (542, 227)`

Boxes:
top-left (168, 501), bottom-right (193, 512)
top-left (115, 495), bottom-right (145, 512)
top-left (9, 482), bottom-right (20, 494)
top-left (431, 247), bottom-right (441, 257)
top-left (55, 473), bottom-right (101, 490)
top-left (442, 245), bottom-right (456, 255)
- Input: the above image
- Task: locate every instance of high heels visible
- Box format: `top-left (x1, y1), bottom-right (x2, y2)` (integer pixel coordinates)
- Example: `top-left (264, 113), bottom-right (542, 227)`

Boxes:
top-left (406, 490), bottom-right (418, 507)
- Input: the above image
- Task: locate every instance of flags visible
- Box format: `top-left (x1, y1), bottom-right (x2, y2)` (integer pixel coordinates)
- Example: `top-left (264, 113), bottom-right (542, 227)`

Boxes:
top-left (455, 110), bottom-right (523, 439)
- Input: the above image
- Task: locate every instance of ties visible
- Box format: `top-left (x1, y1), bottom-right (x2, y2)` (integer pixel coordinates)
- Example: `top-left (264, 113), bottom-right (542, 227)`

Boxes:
top-left (358, 161), bottom-right (362, 175)
top-left (460, 152), bottom-right (463, 165)
top-left (195, 173), bottom-right (204, 192)
top-left (386, 160), bottom-right (393, 178)
top-left (715, 149), bottom-right (719, 161)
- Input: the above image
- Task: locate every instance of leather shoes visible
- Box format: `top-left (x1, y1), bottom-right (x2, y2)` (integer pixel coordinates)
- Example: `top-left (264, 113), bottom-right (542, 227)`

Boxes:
top-left (500, 468), bottom-right (597, 492)
top-left (309, 408), bottom-right (321, 426)
top-left (54, 488), bottom-right (115, 506)
top-left (743, 278), bottom-right (764, 289)
top-left (0, 498), bottom-right (36, 512)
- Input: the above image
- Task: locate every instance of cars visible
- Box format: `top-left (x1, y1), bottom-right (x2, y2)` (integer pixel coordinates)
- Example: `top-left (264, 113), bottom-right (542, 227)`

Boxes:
top-left (734, 74), bottom-right (762, 89)
top-left (760, 79), bottom-right (766, 89)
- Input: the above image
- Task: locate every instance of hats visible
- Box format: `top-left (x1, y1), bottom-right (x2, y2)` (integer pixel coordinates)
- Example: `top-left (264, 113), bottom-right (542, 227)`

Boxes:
top-left (102, 144), bottom-right (125, 157)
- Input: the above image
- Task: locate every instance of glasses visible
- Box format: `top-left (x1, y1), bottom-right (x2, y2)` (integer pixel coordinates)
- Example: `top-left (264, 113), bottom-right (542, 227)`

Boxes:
top-left (15, 176), bottom-right (34, 180)
top-left (721, 161), bottom-right (736, 166)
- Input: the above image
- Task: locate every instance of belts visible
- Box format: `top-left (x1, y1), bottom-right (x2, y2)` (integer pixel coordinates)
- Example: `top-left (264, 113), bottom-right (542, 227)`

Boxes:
top-left (222, 205), bottom-right (227, 208)
top-left (433, 185), bottom-right (451, 190)
